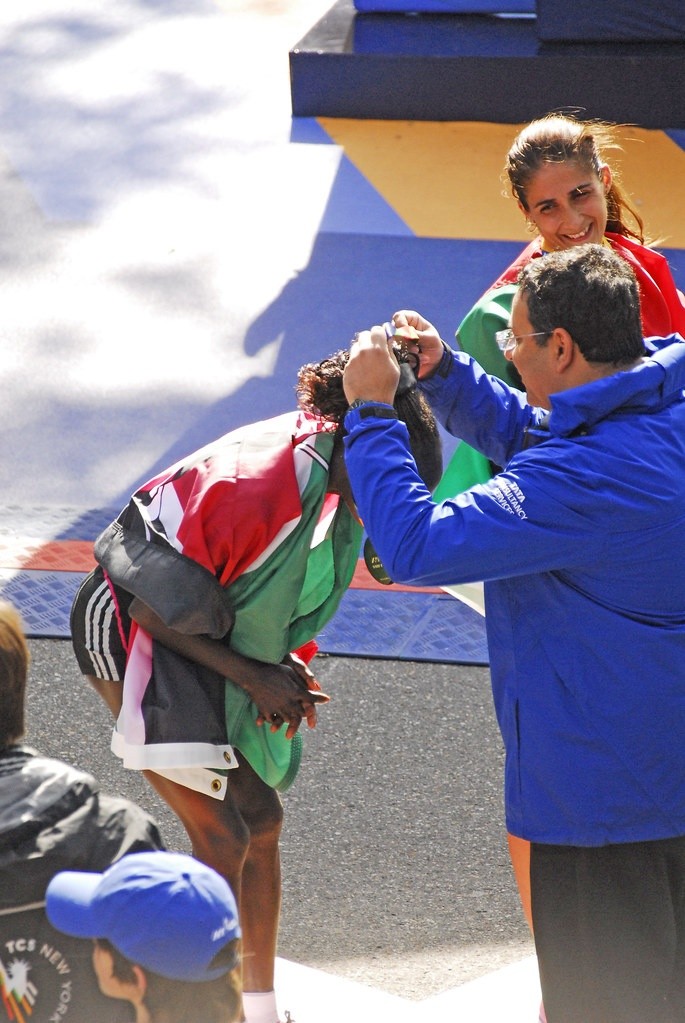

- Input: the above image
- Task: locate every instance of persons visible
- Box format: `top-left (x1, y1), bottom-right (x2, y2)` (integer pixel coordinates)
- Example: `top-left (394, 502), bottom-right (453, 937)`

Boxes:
top-left (69, 331), bottom-right (443, 1023)
top-left (44, 851), bottom-right (249, 1023)
top-left (0, 590), bottom-right (164, 1023)
top-left (340, 107), bottom-right (685, 1023)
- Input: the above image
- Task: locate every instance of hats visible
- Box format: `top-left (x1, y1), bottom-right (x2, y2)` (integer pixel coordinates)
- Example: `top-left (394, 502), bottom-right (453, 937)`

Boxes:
top-left (43, 850), bottom-right (241, 982)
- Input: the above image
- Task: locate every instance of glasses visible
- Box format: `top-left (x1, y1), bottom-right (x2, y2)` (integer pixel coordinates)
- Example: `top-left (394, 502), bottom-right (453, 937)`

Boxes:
top-left (493, 327), bottom-right (557, 350)
